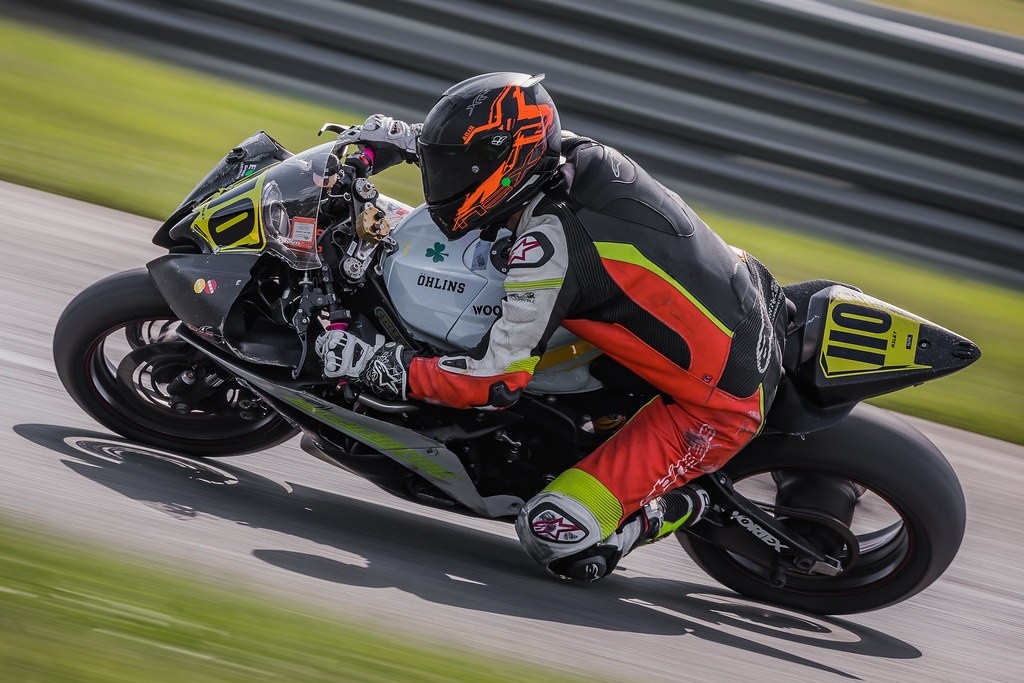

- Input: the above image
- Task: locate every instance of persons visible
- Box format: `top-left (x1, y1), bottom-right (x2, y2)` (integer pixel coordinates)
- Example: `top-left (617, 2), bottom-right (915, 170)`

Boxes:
top-left (315, 72), bottom-right (796, 585)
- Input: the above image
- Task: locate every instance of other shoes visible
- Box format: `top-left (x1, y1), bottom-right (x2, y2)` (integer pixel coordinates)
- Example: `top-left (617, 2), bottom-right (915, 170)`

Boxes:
top-left (679, 487), bottom-right (711, 529)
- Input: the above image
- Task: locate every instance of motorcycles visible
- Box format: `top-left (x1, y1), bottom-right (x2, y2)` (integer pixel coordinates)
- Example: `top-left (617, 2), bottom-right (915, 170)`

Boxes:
top-left (52, 115), bottom-right (982, 617)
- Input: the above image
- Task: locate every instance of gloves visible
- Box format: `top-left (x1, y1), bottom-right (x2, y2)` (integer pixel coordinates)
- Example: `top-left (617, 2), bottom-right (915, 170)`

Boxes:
top-left (337, 112), bottom-right (422, 176)
top-left (316, 329), bottom-right (384, 381)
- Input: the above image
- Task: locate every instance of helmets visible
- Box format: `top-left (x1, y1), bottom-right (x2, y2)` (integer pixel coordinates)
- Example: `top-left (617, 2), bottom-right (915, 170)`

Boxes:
top-left (422, 73), bottom-right (561, 241)
